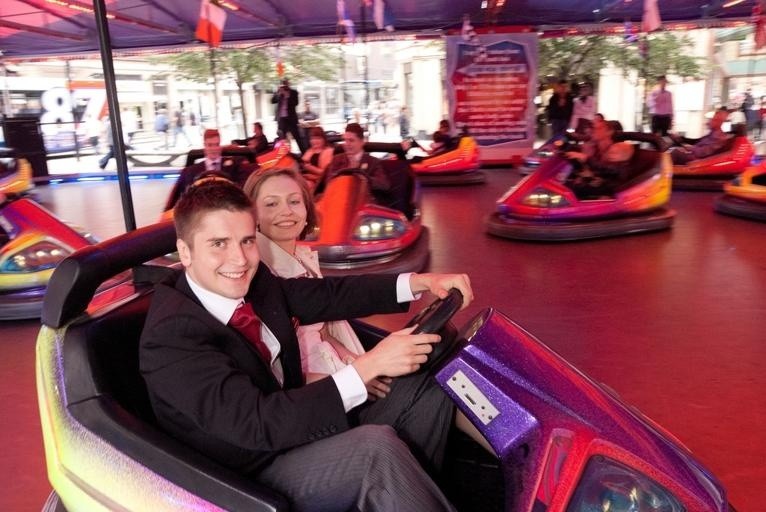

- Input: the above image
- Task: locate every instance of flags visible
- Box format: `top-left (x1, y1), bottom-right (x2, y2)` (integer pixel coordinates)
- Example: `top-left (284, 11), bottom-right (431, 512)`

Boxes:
top-left (370, 1), bottom-right (396, 35)
top-left (193, 2), bottom-right (228, 49)
top-left (337, 1), bottom-right (357, 46)
top-left (641, 0), bottom-right (665, 35)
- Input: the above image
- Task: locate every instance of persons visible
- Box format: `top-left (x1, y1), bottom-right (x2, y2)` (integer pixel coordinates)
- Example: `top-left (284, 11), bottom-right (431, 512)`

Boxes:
top-left (563, 111), bottom-right (638, 199)
top-left (702, 90), bottom-right (766, 139)
top-left (313, 122), bottom-right (390, 197)
top-left (568, 84), bottom-right (599, 131)
top-left (669, 109), bottom-right (731, 165)
top-left (162, 128), bottom-right (248, 212)
top-left (429, 120), bottom-right (458, 155)
top-left (294, 99), bottom-right (319, 148)
top-left (98, 99), bottom-right (198, 169)
top-left (270, 77), bottom-right (307, 154)
top-left (136, 177), bottom-right (475, 512)
top-left (300, 126), bottom-right (335, 175)
top-left (232, 122), bottom-right (270, 155)
top-left (647, 75), bottom-right (675, 135)
top-left (547, 79), bottom-right (574, 136)
top-left (238, 166), bottom-right (500, 477)
top-left (397, 106), bottom-right (411, 141)
